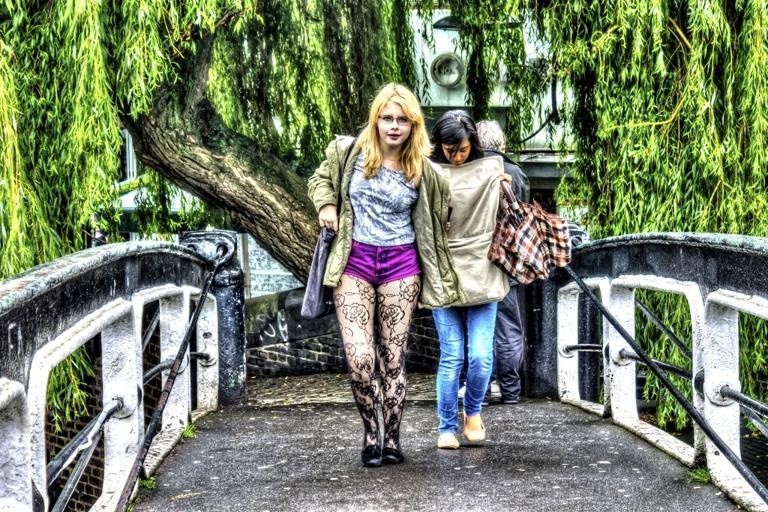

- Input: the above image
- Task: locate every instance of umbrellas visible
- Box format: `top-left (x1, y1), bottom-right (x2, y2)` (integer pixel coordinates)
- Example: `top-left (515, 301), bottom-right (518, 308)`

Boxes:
top-left (489, 178), bottom-right (573, 287)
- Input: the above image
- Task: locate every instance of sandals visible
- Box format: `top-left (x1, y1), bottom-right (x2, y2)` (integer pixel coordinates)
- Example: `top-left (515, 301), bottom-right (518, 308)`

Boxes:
top-left (438, 432), bottom-right (459, 449)
top-left (463, 410), bottom-right (485, 442)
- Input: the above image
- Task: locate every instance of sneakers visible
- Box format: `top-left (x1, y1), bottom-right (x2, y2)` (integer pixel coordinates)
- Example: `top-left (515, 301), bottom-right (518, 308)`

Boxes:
top-left (481, 397), bottom-right (518, 405)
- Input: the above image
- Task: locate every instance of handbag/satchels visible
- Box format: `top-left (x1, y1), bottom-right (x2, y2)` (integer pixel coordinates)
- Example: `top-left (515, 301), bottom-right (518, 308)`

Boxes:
top-left (300, 226), bottom-right (336, 320)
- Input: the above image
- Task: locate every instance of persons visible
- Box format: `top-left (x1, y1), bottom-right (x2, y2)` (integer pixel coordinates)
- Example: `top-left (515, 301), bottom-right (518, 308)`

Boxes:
top-left (478, 118), bottom-right (533, 407)
top-left (304, 81), bottom-right (463, 469)
top-left (415, 111), bottom-right (514, 451)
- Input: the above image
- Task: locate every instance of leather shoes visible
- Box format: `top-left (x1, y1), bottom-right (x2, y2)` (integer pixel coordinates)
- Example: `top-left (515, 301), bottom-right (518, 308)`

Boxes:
top-left (361, 444), bottom-right (401, 467)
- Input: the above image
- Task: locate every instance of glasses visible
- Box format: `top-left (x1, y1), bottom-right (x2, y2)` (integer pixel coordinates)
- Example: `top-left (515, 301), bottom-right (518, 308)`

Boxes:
top-left (378, 114), bottom-right (409, 126)
